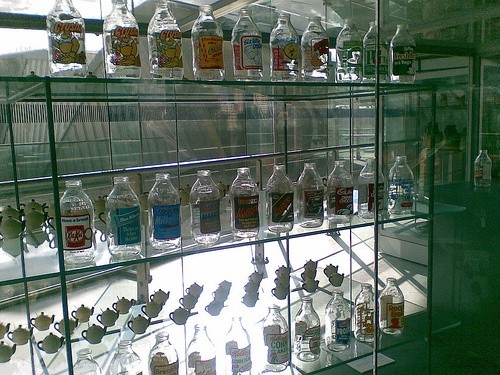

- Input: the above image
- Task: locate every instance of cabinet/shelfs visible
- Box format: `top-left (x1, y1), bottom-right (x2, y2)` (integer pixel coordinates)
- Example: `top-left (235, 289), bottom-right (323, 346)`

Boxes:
top-left (0, 0), bottom-right (439, 375)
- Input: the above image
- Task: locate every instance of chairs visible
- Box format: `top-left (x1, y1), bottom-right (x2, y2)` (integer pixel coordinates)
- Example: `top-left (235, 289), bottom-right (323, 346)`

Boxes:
top-left (451, 186), bottom-right (500, 313)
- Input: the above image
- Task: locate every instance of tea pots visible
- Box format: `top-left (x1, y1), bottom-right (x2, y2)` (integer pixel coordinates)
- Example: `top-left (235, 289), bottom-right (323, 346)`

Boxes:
top-left (0, 182), bottom-right (260, 238)
top-left (0, 259), bottom-right (345, 363)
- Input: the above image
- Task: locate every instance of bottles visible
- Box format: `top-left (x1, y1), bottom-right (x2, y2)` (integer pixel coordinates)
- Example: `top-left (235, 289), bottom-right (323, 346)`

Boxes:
top-left (358, 157), bottom-right (384, 219)
top-left (388, 156), bottom-right (414, 216)
top-left (105, 177), bottom-right (143, 258)
top-left (102, 0), bottom-right (142, 78)
top-left (147, 173), bottom-right (181, 250)
top-left (190, 4), bottom-right (263, 80)
top-left (46, 0), bottom-right (88, 77)
top-left (326, 161), bottom-right (354, 223)
top-left (297, 163), bottom-right (324, 228)
top-left (269, 13), bottom-right (329, 82)
top-left (60, 178), bottom-right (95, 263)
top-left (72, 278), bottom-right (405, 375)
top-left (474, 150), bottom-right (491, 186)
top-left (147, 0), bottom-right (185, 80)
top-left (265, 165), bottom-right (293, 233)
top-left (229, 168), bottom-right (259, 238)
top-left (189, 170), bottom-right (221, 244)
top-left (334, 18), bottom-right (417, 83)
